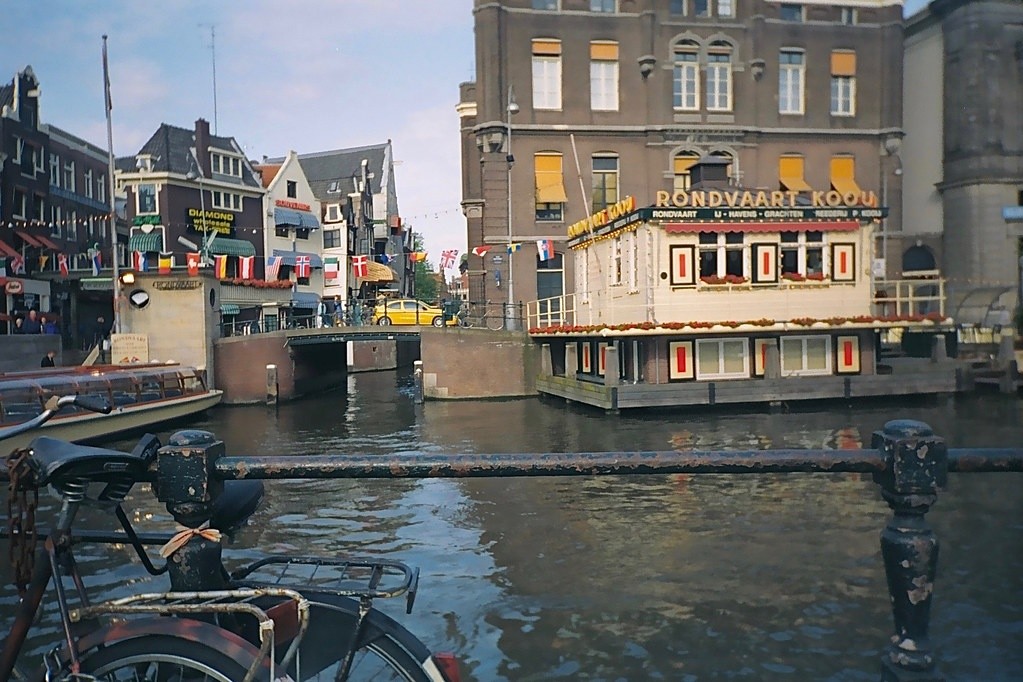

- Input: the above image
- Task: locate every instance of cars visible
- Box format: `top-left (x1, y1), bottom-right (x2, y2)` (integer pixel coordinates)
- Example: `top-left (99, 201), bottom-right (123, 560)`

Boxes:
top-left (371, 298), bottom-right (460, 327)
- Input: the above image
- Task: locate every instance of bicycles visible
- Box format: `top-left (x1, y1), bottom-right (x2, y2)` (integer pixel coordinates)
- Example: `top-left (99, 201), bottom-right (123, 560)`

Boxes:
top-left (288, 304), bottom-right (378, 327)
top-left (456, 299), bottom-right (505, 331)
top-left (0, 396), bottom-right (458, 682)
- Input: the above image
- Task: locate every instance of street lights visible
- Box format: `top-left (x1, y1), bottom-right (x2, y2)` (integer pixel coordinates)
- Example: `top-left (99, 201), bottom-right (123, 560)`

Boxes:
top-left (505, 84), bottom-right (521, 330)
top-left (185, 162), bottom-right (210, 268)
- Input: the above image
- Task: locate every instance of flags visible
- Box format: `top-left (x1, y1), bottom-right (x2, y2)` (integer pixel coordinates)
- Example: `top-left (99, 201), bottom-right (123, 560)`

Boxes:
top-left (11, 239), bottom-right (555, 281)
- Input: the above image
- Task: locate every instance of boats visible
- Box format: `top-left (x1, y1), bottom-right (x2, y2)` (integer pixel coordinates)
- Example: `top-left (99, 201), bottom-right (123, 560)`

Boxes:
top-left (0, 358), bottom-right (224, 466)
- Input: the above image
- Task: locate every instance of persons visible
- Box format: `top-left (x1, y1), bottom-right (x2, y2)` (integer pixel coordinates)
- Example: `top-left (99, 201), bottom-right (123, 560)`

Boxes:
top-left (440, 299), bottom-right (448, 327)
top-left (88, 312), bottom-right (109, 364)
top-left (41, 351), bottom-right (55, 368)
top-left (12, 310), bottom-right (58, 335)
top-left (316, 295), bottom-right (361, 328)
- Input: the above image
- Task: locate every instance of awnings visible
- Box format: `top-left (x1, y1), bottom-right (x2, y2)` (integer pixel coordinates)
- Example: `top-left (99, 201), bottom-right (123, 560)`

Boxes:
top-left (293, 292), bottom-right (323, 309)
top-left (0, 239), bottom-right (23, 264)
top-left (34, 234), bottom-right (58, 254)
top-left (275, 208), bottom-right (319, 232)
top-left (356, 259), bottom-right (399, 284)
top-left (273, 249), bottom-right (323, 270)
top-left (830, 155), bottom-right (863, 196)
top-left (201, 237), bottom-right (257, 258)
top-left (220, 304), bottom-right (240, 315)
top-left (129, 234), bottom-right (162, 253)
top-left (14, 231), bottom-right (43, 249)
top-left (534, 152), bottom-right (567, 203)
top-left (779, 153), bottom-right (812, 193)
